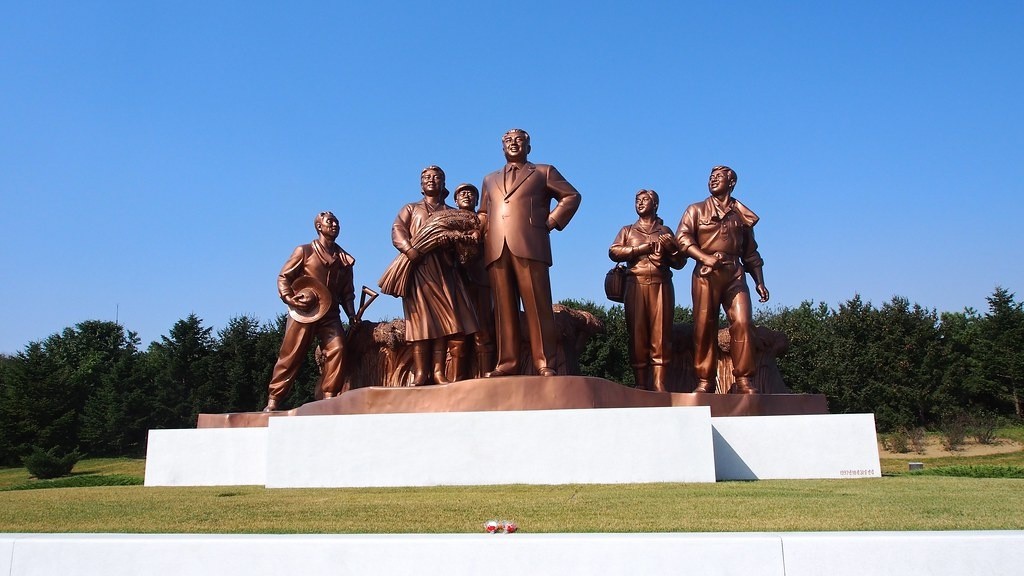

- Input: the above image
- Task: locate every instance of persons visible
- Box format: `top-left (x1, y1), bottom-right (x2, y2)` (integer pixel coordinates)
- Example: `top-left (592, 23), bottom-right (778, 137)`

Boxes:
top-left (674, 166), bottom-right (769, 394)
top-left (461, 129), bottom-right (581, 378)
top-left (609, 190), bottom-right (687, 393)
top-left (448, 182), bottom-right (493, 382)
top-left (392, 165), bottom-right (459, 386)
top-left (263, 212), bottom-right (362, 413)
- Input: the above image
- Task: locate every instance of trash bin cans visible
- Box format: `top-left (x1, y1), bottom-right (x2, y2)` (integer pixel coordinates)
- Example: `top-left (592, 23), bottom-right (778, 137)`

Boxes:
top-left (909, 462), bottom-right (924, 471)
